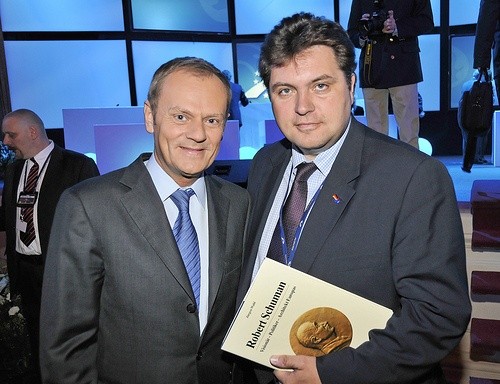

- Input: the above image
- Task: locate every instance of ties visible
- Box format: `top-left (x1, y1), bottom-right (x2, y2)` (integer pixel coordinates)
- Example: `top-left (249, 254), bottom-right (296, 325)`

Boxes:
top-left (20, 157), bottom-right (39, 247)
top-left (265, 163), bottom-right (318, 265)
top-left (170, 188), bottom-right (201, 311)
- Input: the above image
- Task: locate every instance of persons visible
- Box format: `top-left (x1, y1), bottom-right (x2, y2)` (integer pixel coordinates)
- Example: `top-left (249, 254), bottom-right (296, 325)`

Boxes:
top-left (39, 57), bottom-right (252, 384)
top-left (234, 12), bottom-right (472, 384)
top-left (0, 109), bottom-right (101, 384)
top-left (346, 0), bottom-right (434, 150)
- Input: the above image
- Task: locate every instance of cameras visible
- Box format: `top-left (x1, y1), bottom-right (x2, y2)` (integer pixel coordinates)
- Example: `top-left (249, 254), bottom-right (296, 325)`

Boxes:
top-left (359, 0), bottom-right (388, 36)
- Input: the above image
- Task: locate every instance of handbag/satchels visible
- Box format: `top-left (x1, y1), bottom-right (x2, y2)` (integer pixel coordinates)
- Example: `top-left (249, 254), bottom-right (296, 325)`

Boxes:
top-left (460, 67), bottom-right (492, 134)
